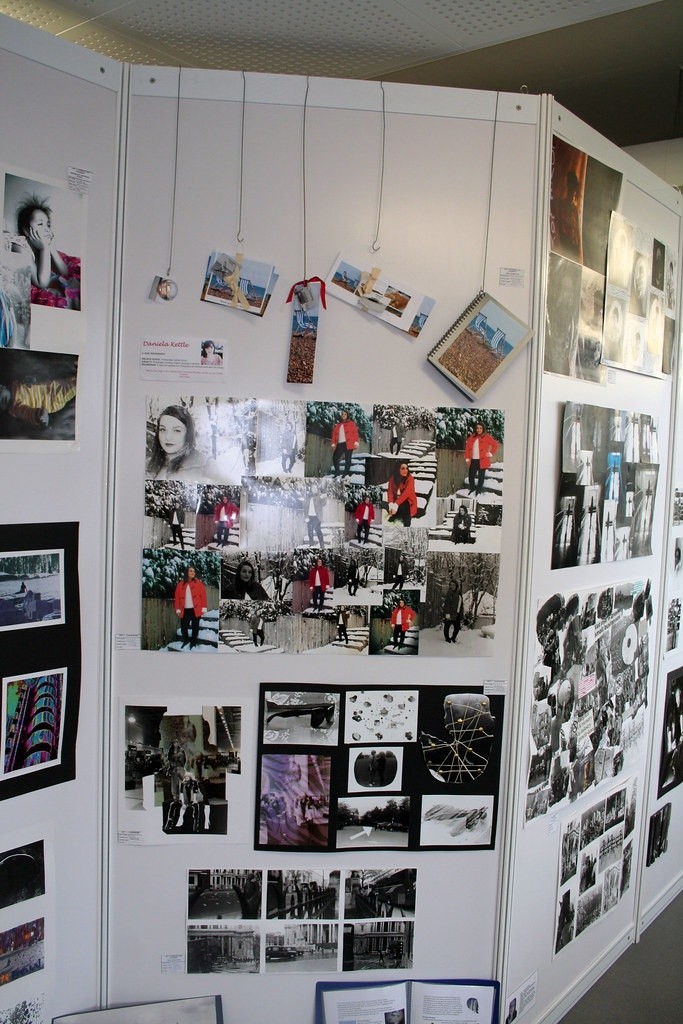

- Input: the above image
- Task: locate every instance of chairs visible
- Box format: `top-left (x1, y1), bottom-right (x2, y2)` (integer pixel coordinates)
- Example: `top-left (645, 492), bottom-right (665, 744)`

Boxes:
top-left (415, 313), bottom-right (428, 329)
top-left (354, 279), bottom-right (359, 290)
top-left (468, 312), bottom-right (487, 342)
top-left (212, 275), bottom-right (257, 302)
top-left (379, 941), bottom-right (403, 968)
top-left (484, 328), bottom-right (506, 363)
top-left (294, 309), bottom-right (317, 336)
top-left (339, 271), bottom-right (350, 287)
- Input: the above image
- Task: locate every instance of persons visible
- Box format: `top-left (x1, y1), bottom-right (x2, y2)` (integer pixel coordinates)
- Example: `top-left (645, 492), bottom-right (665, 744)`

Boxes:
top-left (667, 598), bottom-right (681, 650)
top-left (126, 739), bottom-right (241, 829)
top-left (506, 1002), bottom-right (517, 1024)
top-left (244, 874), bottom-right (260, 904)
top-left (144, 405), bottom-right (497, 651)
top-left (675, 547), bottom-right (681, 572)
top-left (388, 1011), bottom-right (403, 1024)
top-left (523, 578), bottom-right (654, 822)
top-left (3, 193), bottom-right (67, 289)
top-left (671, 688), bottom-right (683, 744)
top-left (556, 803), bottom-right (636, 954)
top-left (14, 582), bottom-right (27, 594)
top-left (201, 341), bottom-right (221, 366)
top-left (260, 792), bottom-right (330, 833)
top-left (0, 273), bottom-right (17, 349)
top-left (377, 950), bottom-right (384, 964)
top-left (549, 171), bottom-right (678, 384)
